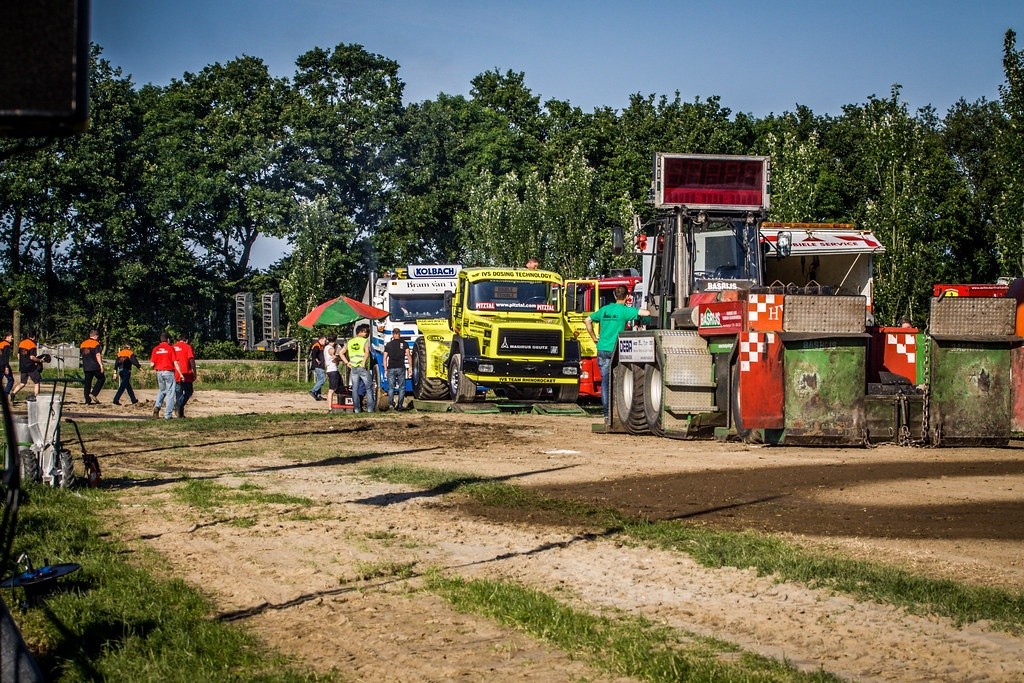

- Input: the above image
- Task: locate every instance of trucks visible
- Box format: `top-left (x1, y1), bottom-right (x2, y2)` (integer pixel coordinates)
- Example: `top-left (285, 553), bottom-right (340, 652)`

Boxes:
top-left (629, 219), bottom-right (890, 416)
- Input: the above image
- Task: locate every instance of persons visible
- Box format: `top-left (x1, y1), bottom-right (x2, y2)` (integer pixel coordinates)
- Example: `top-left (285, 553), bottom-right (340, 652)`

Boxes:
top-left (79, 329), bottom-right (106, 405)
top-left (9, 332), bottom-right (46, 404)
top-left (382, 328), bottom-right (412, 411)
top-left (171, 333), bottom-right (197, 419)
top-left (308, 336), bottom-right (327, 401)
top-left (323, 334), bottom-right (346, 412)
top-left (583, 284), bottom-right (650, 423)
top-left (110, 341), bottom-right (145, 406)
top-left (0, 332), bottom-right (14, 405)
top-left (149, 332), bottom-right (186, 419)
top-left (338, 323), bottom-right (376, 413)
top-left (521, 258), bottom-right (540, 269)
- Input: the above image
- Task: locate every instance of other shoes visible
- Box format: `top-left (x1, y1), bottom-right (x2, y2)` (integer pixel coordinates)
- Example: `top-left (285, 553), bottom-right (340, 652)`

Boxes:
top-left (113, 400), bottom-right (122, 405)
top-left (132, 399), bottom-right (138, 404)
top-left (309, 390), bottom-right (319, 401)
top-left (175, 410), bottom-right (185, 418)
top-left (10, 393), bottom-right (16, 405)
top-left (152, 407), bottom-right (160, 419)
top-left (328, 409), bottom-right (332, 412)
top-left (85, 394), bottom-right (100, 405)
top-left (315, 395), bottom-right (327, 400)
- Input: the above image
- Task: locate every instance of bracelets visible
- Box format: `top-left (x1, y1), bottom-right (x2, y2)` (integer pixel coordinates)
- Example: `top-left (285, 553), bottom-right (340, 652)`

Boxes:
top-left (39, 359), bottom-right (42, 362)
top-left (100, 365), bottom-right (103, 367)
top-left (114, 373), bottom-right (117, 374)
top-left (384, 370), bottom-right (387, 371)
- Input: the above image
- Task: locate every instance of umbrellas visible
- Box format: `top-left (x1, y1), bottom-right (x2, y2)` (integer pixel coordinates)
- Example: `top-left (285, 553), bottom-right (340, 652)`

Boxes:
top-left (296, 296), bottom-right (391, 338)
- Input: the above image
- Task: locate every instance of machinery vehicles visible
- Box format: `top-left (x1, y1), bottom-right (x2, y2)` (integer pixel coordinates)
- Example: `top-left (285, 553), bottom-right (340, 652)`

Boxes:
top-left (593, 148), bottom-right (793, 446)
top-left (352, 259), bottom-right (644, 403)
